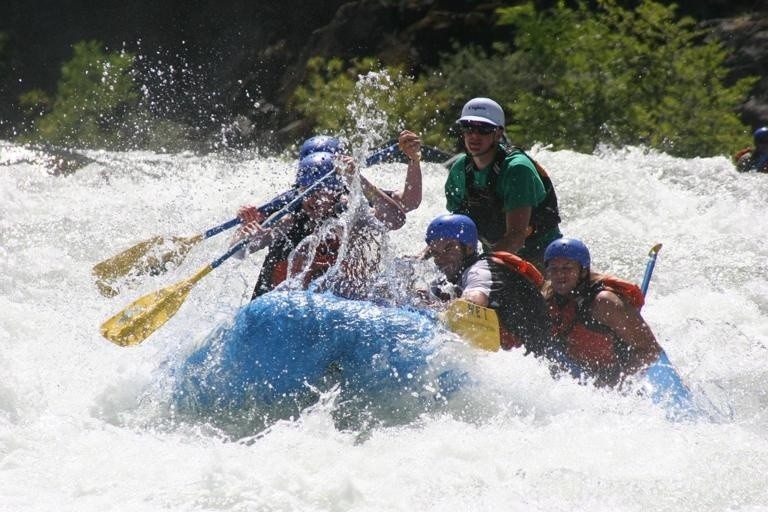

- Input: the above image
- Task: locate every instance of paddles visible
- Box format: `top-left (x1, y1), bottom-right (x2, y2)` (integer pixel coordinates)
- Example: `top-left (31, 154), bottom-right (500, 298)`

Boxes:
top-left (97, 160), bottom-right (350, 347)
top-left (396, 298), bottom-right (502, 354)
top-left (92, 140), bottom-right (401, 298)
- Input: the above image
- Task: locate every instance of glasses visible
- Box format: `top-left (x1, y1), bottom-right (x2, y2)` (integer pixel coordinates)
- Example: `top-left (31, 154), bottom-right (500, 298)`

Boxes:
top-left (459, 122), bottom-right (497, 136)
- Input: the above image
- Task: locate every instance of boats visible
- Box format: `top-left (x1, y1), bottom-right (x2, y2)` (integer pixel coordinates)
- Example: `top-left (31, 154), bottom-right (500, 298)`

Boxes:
top-left (174, 292), bottom-right (689, 417)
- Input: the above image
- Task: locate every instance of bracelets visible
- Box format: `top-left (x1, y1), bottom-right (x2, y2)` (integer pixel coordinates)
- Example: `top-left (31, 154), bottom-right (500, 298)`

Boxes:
top-left (408, 150), bottom-right (424, 163)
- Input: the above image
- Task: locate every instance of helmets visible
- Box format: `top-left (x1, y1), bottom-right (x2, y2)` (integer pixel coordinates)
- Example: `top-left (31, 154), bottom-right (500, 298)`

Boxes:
top-left (297, 136), bottom-right (344, 188)
top-left (425, 214), bottom-right (477, 245)
top-left (543, 238), bottom-right (590, 270)
top-left (456, 97), bottom-right (505, 128)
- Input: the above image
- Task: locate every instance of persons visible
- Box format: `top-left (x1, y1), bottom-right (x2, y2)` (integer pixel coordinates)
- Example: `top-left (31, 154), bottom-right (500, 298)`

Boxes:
top-left (443, 96), bottom-right (562, 280)
top-left (540, 237), bottom-right (663, 395)
top-left (229, 127), bottom-right (425, 230)
top-left (732, 126), bottom-right (768, 172)
top-left (224, 152), bottom-right (408, 300)
top-left (422, 212), bottom-right (552, 355)
